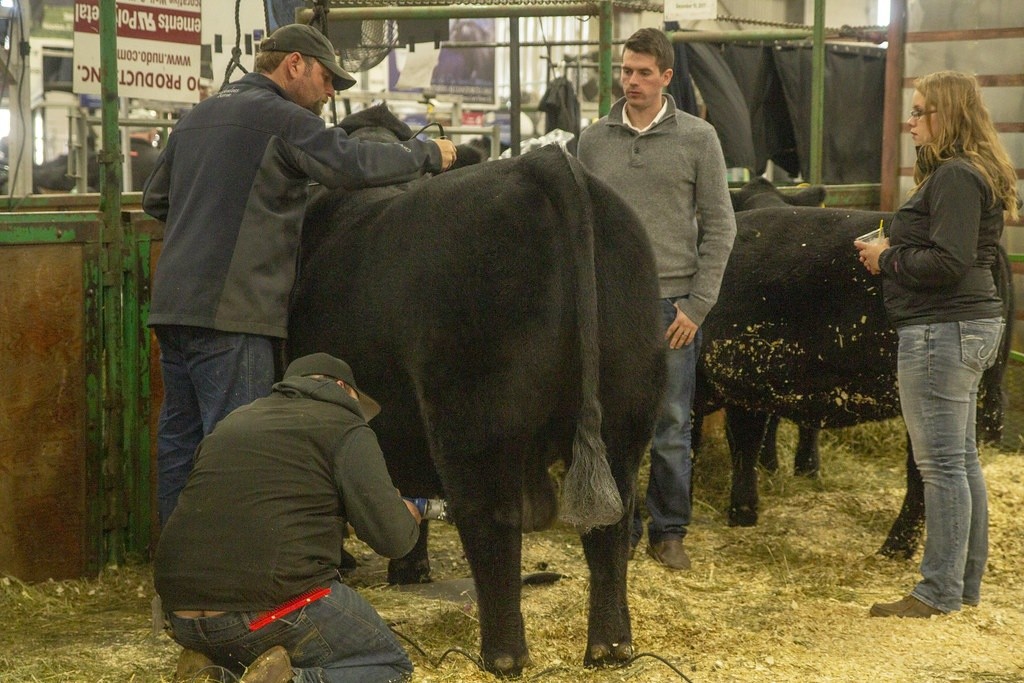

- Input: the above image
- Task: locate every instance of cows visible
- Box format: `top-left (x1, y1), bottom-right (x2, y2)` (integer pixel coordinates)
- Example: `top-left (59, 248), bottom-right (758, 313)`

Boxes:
top-left (691, 177), bottom-right (1014, 562)
top-left (277, 98), bottom-right (665, 679)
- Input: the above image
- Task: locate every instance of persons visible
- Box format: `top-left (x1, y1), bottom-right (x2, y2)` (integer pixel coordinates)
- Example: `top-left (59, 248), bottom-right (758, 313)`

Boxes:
top-left (142, 22), bottom-right (456, 550)
top-left (127, 111), bottom-right (170, 192)
top-left (0, 135), bottom-right (13, 194)
top-left (154, 352), bottom-right (425, 683)
top-left (577, 26), bottom-right (737, 569)
top-left (856, 68), bottom-right (1021, 617)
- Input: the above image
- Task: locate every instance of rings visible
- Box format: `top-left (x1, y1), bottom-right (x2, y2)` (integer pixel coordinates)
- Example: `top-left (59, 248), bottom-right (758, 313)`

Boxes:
top-left (682, 332), bottom-right (689, 336)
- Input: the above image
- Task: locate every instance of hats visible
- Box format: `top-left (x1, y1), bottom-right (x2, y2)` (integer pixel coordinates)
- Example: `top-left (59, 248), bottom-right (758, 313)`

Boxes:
top-left (282, 352), bottom-right (381, 424)
top-left (261, 24), bottom-right (357, 91)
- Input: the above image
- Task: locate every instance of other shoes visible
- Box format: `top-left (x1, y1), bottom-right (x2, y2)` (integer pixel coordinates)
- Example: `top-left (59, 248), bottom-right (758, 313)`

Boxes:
top-left (175, 648), bottom-right (217, 682)
top-left (870, 593), bottom-right (941, 619)
top-left (629, 534), bottom-right (639, 560)
top-left (238, 645), bottom-right (296, 682)
top-left (646, 539), bottom-right (691, 570)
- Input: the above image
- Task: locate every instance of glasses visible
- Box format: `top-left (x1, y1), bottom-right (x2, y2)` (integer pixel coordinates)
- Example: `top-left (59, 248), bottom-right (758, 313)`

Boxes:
top-left (909, 108), bottom-right (936, 119)
